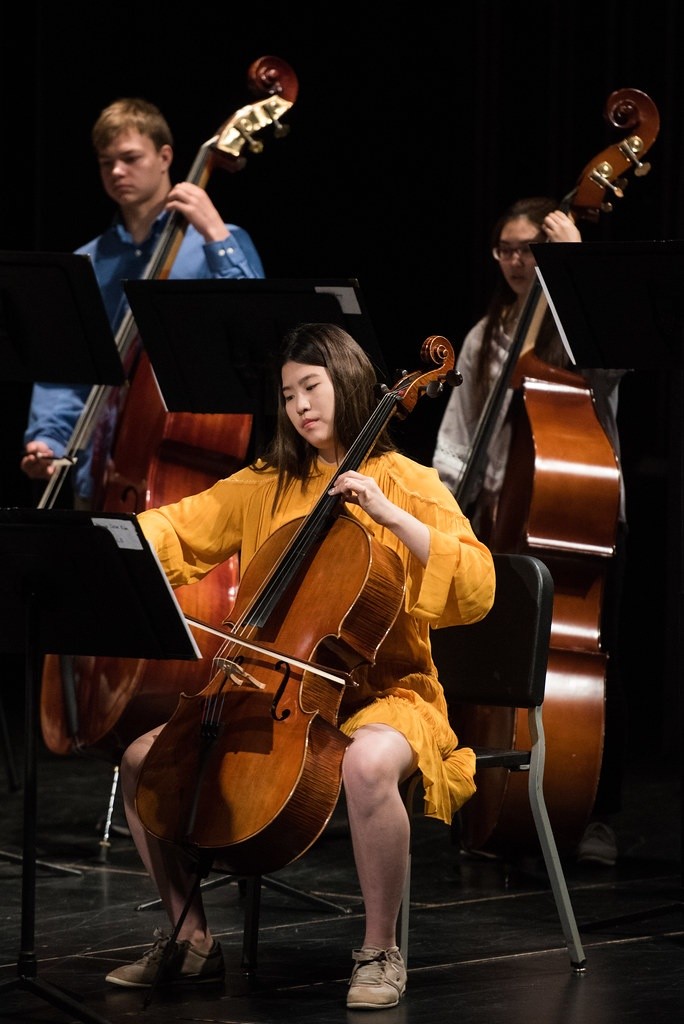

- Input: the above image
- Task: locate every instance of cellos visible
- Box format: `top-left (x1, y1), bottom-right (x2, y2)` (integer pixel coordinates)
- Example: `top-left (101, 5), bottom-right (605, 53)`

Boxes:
top-left (35, 51), bottom-right (303, 866)
top-left (452, 86), bottom-right (667, 840)
top-left (138, 332), bottom-right (463, 1015)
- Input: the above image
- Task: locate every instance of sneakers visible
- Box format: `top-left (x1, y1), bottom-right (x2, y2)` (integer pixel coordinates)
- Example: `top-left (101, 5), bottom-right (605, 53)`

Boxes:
top-left (104, 928), bottom-right (224, 988)
top-left (346, 947), bottom-right (407, 1008)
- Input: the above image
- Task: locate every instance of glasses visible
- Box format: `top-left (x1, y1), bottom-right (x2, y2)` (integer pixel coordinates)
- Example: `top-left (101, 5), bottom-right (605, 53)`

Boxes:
top-left (492, 245), bottom-right (533, 260)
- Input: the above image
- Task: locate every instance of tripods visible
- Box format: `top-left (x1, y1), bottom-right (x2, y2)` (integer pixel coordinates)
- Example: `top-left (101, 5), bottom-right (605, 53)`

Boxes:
top-left (0, 504), bottom-right (202, 1024)
top-left (122, 278), bottom-right (358, 920)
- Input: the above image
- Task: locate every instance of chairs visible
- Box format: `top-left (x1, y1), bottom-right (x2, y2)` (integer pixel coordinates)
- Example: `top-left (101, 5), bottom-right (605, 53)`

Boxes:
top-left (237, 554), bottom-right (586, 983)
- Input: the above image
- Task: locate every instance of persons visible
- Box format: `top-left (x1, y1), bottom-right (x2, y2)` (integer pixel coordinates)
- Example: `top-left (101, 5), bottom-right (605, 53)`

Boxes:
top-left (102, 322), bottom-right (498, 1009)
top-left (432, 200), bottom-right (628, 869)
top-left (21, 99), bottom-right (266, 836)
